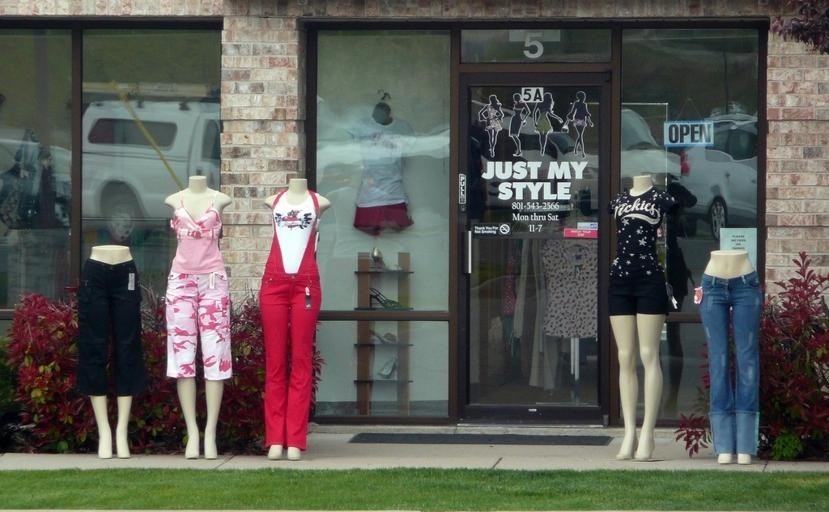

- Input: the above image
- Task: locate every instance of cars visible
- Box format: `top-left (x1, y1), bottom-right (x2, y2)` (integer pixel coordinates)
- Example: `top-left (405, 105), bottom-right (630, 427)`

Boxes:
top-left (0, 138), bottom-right (70, 225)
top-left (477, 114), bottom-right (600, 217)
top-left (676, 115), bottom-right (762, 242)
top-left (620, 107), bottom-right (683, 196)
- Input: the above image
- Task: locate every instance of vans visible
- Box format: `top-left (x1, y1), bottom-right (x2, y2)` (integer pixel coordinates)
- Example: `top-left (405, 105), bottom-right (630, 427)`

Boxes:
top-left (82, 99), bottom-right (220, 243)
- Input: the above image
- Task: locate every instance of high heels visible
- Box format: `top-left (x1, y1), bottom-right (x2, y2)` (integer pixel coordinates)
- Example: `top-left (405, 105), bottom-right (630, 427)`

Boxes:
top-left (365, 325), bottom-right (402, 346)
top-left (370, 245), bottom-right (404, 272)
top-left (374, 356), bottom-right (398, 380)
top-left (366, 286), bottom-right (415, 312)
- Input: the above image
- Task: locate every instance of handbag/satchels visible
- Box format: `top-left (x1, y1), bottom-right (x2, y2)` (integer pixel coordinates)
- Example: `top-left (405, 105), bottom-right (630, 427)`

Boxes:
top-left (0, 142), bottom-right (66, 231)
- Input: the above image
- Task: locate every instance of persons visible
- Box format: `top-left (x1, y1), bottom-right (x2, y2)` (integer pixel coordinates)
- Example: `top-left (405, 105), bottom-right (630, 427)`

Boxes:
top-left (75, 244), bottom-right (146, 457)
top-left (161, 176), bottom-right (237, 458)
top-left (258, 177), bottom-right (333, 461)
top-left (342, 101), bottom-right (415, 237)
top-left (699, 249), bottom-right (763, 464)
top-left (604, 174), bottom-right (672, 461)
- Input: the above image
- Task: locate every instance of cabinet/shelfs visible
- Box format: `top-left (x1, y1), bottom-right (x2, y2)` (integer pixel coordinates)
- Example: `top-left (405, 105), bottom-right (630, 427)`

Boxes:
top-left (350, 249), bottom-right (416, 418)
